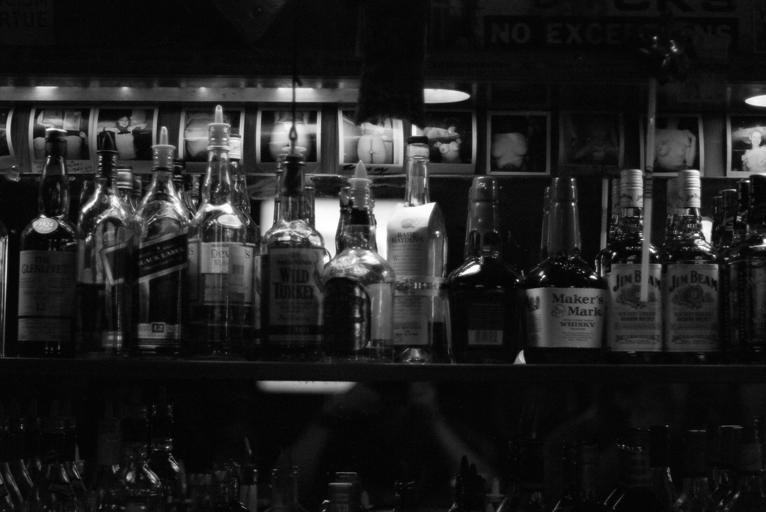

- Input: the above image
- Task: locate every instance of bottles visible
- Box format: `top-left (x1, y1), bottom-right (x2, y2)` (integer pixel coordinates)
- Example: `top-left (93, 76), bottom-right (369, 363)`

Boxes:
top-left (0, 384), bottom-right (766, 512)
top-left (15, 101), bottom-right (766, 363)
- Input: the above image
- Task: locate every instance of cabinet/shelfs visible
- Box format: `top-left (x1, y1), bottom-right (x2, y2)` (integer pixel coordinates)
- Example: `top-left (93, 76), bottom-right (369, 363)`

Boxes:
top-left (0, 78), bottom-right (766, 385)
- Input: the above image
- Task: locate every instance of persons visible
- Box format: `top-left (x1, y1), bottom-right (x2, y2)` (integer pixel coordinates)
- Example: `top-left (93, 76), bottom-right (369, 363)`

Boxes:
top-left (30, 112), bottom-right (766, 176)
